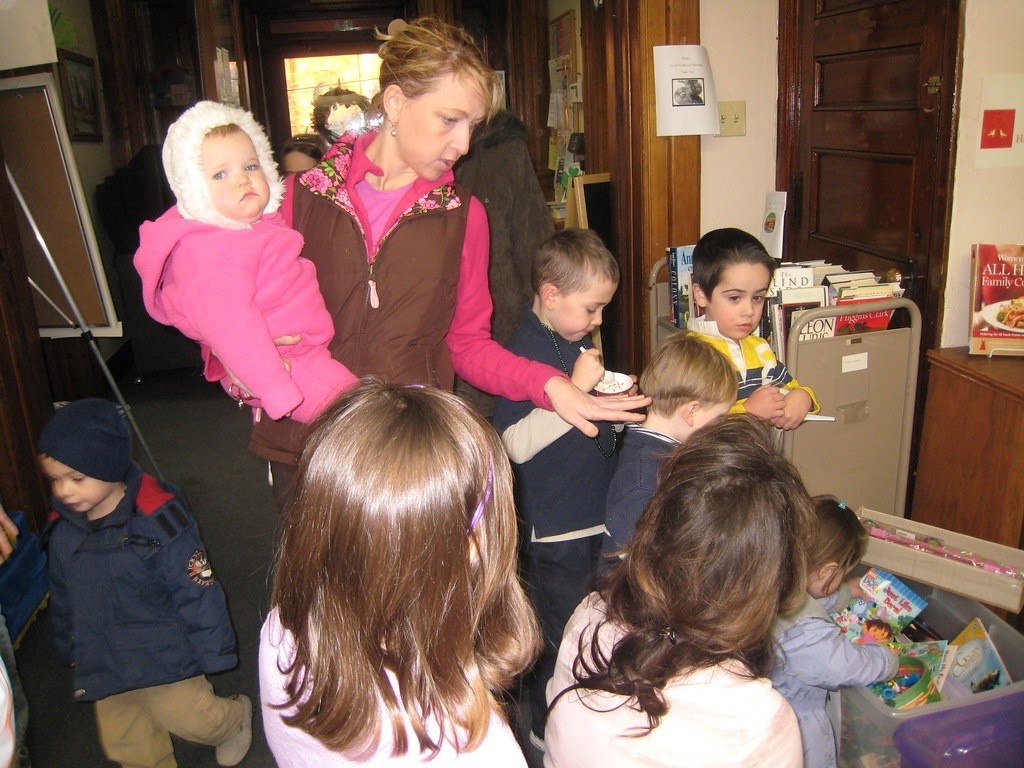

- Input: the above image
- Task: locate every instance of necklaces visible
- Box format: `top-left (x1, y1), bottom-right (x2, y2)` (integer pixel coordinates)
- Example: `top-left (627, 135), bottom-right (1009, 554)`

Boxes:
top-left (544, 325), bottom-right (617, 457)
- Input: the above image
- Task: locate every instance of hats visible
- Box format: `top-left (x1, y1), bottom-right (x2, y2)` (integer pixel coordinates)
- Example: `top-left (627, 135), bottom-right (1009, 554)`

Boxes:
top-left (165, 101), bottom-right (282, 228)
top-left (38, 399), bottom-right (132, 482)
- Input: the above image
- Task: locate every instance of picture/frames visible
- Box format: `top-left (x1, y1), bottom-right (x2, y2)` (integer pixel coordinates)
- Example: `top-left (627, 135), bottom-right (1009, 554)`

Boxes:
top-left (56, 48), bottom-right (103, 143)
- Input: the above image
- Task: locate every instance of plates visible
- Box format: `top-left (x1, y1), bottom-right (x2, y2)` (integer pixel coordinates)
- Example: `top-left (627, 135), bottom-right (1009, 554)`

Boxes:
top-left (981, 300), bottom-right (1024, 334)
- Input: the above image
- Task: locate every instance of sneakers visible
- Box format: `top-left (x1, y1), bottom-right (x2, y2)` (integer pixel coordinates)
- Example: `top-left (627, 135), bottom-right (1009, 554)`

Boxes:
top-left (216, 693), bottom-right (253, 766)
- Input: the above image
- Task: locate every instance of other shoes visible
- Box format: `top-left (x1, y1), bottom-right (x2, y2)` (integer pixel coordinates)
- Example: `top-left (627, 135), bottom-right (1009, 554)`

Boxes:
top-left (529, 725), bottom-right (547, 752)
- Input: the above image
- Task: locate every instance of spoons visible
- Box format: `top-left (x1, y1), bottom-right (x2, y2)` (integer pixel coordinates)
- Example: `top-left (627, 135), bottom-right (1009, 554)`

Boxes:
top-left (580, 347), bottom-right (615, 384)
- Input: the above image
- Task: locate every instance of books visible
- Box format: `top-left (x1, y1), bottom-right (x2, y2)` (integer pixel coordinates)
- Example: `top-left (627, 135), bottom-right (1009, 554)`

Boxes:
top-left (967, 244), bottom-right (1024, 356)
top-left (665, 243), bottom-right (905, 369)
top-left (827, 566), bottom-right (1012, 711)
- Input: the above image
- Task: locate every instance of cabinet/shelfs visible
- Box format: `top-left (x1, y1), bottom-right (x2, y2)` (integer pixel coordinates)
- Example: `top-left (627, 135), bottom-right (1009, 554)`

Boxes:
top-left (912, 345), bottom-right (1024, 636)
top-left (650, 257), bottom-right (922, 518)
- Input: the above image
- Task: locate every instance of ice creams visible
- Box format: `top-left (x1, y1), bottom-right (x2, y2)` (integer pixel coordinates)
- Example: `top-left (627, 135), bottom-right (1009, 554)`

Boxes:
top-left (596, 378), bottom-right (625, 392)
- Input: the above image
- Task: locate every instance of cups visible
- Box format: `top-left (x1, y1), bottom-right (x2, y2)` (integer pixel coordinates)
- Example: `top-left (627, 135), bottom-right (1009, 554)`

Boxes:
top-left (593, 371), bottom-right (633, 396)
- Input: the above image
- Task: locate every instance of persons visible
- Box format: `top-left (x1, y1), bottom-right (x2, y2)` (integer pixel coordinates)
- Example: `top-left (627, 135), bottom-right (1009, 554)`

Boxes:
top-left (595, 330), bottom-right (738, 591)
top-left (94, 144), bottom-right (206, 374)
top-left (685, 227), bottom-right (820, 431)
top-left (543, 412), bottom-right (818, 768)
top-left (456, 110), bottom-right (558, 583)
top-left (279, 132), bottom-right (328, 173)
top-left (494, 228), bottom-right (639, 753)
top-left (132, 100), bottom-right (361, 423)
top-left (34, 398), bottom-right (252, 768)
top-left (257, 373), bottom-right (543, 768)
top-left (199, 15), bottom-right (652, 518)
top-left (761, 493), bottom-right (899, 768)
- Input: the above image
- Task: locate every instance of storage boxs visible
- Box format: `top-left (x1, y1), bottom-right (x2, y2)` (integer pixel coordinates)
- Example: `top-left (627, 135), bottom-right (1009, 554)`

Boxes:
top-left (852, 506), bottom-right (1024, 616)
top-left (835, 563), bottom-right (1024, 768)
